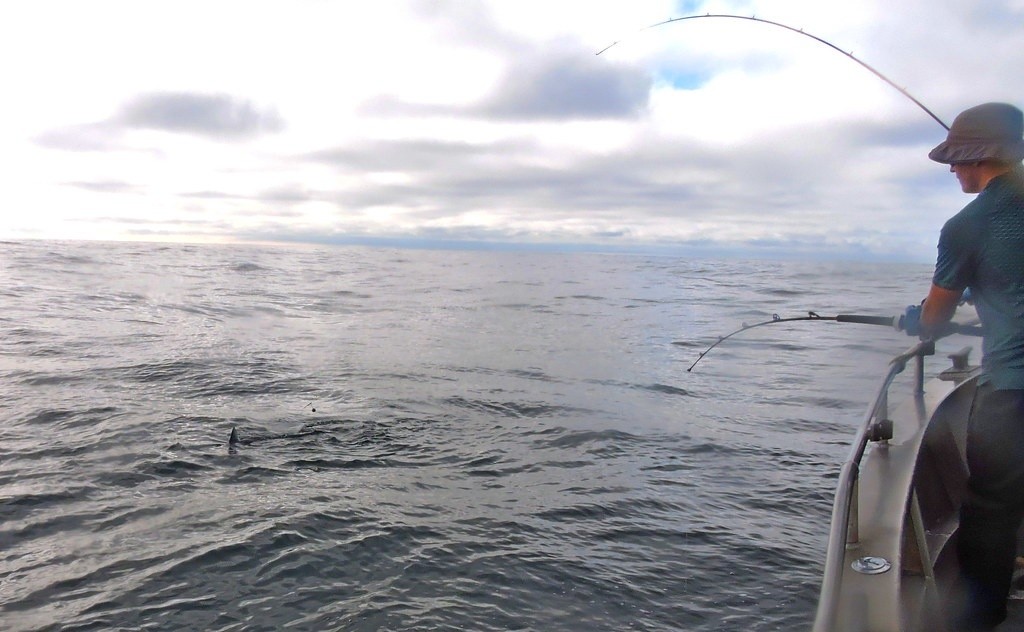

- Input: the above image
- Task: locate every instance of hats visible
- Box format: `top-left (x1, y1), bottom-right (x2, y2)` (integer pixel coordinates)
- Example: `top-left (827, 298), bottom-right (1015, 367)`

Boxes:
top-left (928, 102), bottom-right (1024, 164)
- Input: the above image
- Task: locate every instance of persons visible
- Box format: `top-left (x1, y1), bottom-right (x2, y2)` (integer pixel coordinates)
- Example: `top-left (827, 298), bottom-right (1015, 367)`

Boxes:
top-left (919, 102), bottom-right (1024, 632)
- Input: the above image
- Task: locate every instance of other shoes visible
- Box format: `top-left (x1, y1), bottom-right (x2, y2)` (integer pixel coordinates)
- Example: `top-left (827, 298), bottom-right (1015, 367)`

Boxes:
top-left (968, 603), bottom-right (1007, 630)
top-left (1013, 575), bottom-right (1024, 590)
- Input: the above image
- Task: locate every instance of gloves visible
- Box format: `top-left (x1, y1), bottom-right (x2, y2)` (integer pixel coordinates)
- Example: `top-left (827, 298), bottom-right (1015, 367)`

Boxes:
top-left (959, 288), bottom-right (973, 305)
top-left (904, 305), bottom-right (922, 336)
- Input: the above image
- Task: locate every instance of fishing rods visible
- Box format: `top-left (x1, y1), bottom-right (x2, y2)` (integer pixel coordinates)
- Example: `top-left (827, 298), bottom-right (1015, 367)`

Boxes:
top-left (594, 11), bottom-right (950, 130)
top-left (687, 307), bottom-right (983, 370)
